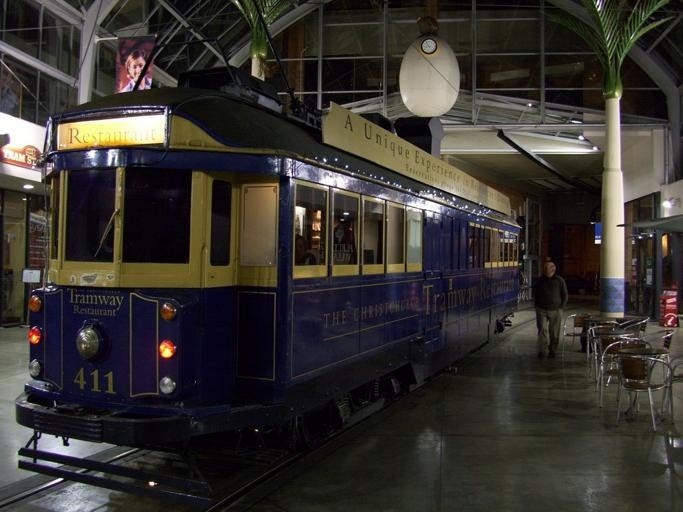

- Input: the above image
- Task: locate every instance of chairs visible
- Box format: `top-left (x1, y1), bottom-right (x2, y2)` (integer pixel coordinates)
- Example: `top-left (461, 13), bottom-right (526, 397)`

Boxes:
top-left (559, 310), bottom-right (681, 434)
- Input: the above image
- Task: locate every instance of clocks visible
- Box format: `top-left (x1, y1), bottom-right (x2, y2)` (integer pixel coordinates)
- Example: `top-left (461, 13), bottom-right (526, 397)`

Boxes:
top-left (420, 38), bottom-right (438, 55)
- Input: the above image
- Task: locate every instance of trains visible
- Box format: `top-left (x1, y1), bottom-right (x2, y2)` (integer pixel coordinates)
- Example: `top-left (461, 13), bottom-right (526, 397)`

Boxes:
top-left (14, 66), bottom-right (525, 509)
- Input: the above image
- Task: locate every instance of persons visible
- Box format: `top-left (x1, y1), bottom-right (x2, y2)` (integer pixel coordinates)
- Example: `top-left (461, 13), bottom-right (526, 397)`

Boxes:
top-left (534, 261), bottom-right (569, 360)
top-left (118, 49), bottom-right (152, 94)
top-left (294, 233), bottom-right (311, 265)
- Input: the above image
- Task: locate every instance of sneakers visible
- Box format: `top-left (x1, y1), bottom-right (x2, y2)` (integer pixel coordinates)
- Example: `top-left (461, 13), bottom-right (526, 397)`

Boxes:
top-left (548, 352), bottom-right (555, 358)
top-left (538, 352), bottom-right (544, 358)
